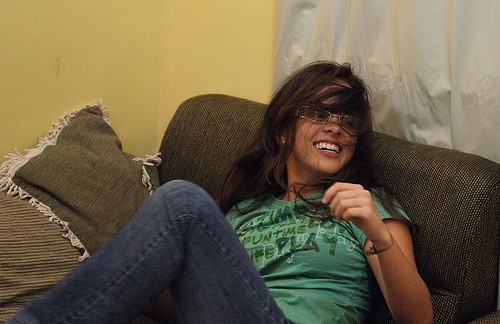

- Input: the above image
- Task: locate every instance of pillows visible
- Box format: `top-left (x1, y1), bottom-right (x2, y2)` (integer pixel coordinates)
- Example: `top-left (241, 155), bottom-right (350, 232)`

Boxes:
top-left (0, 99), bottom-right (162, 262)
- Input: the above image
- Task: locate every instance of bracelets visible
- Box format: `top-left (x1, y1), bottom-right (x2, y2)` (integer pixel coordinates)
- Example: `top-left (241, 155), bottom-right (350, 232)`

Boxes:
top-left (366, 234), bottom-right (394, 256)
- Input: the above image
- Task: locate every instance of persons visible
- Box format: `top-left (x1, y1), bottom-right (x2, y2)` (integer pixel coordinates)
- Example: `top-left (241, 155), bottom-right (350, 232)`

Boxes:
top-left (6, 60), bottom-right (433, 323)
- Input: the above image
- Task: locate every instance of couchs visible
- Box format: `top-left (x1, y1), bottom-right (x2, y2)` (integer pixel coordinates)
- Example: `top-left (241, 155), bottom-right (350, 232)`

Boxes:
top-left (0, 94), bottom-right (500, 324)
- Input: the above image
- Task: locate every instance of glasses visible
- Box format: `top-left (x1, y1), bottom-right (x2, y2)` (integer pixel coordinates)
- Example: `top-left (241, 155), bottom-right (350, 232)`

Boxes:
top-left (290, 106), bottom-right (365, 133)
